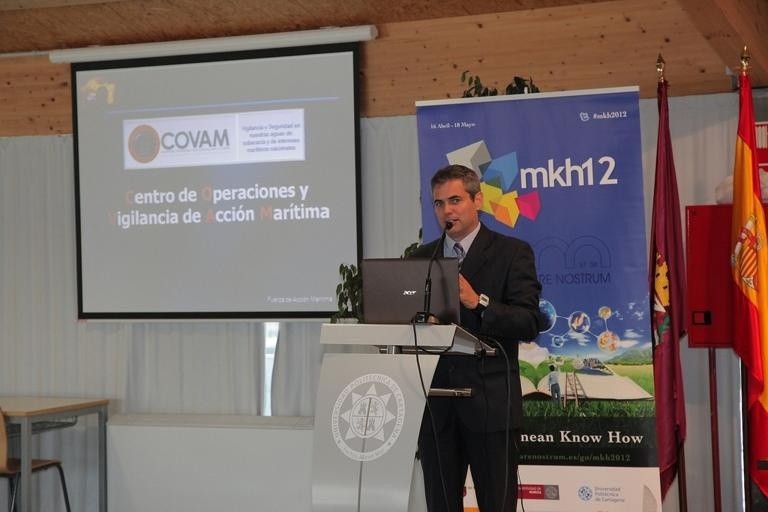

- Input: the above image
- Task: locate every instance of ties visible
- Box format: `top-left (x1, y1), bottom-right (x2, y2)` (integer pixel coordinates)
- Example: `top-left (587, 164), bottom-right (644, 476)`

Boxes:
top-left (453, 243), bottom-right (464, 271)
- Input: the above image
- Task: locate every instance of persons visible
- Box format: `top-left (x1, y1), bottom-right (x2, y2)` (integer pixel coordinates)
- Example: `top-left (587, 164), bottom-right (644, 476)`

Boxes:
top-left (402, 164), bottom-right (543, 512)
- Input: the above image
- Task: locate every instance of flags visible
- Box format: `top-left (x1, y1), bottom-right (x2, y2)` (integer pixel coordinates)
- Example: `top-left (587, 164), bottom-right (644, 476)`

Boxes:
top-left (644, 80), bottom-right (689, 507)
top-left (730, 61), bottom-right (768, 499)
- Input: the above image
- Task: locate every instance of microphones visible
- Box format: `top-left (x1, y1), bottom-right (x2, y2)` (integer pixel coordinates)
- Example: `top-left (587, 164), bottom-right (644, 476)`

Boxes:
top-left (411, 222), bottom-right (453, 325)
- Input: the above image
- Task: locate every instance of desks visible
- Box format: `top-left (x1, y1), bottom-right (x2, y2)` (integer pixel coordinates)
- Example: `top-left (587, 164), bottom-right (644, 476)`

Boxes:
top-left (0, 393), bottom-right (112, 511)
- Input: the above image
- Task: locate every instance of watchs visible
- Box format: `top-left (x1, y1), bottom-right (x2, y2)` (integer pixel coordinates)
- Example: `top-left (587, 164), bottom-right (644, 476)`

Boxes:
top-left (471, 291), bottom-right (490, 317)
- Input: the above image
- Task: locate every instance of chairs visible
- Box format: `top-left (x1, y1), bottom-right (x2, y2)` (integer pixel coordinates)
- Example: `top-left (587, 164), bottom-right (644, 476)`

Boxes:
top-left (2, 404), bottom-right (71, 510)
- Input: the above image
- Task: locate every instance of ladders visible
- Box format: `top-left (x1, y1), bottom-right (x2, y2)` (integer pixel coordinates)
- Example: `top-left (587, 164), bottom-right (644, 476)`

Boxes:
top-left (564, 370), bottom-right (587, 407)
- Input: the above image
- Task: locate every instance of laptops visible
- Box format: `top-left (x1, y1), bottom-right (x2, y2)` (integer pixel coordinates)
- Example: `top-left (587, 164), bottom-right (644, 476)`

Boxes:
top-left (361, 258), bottom-right (461, 326)
